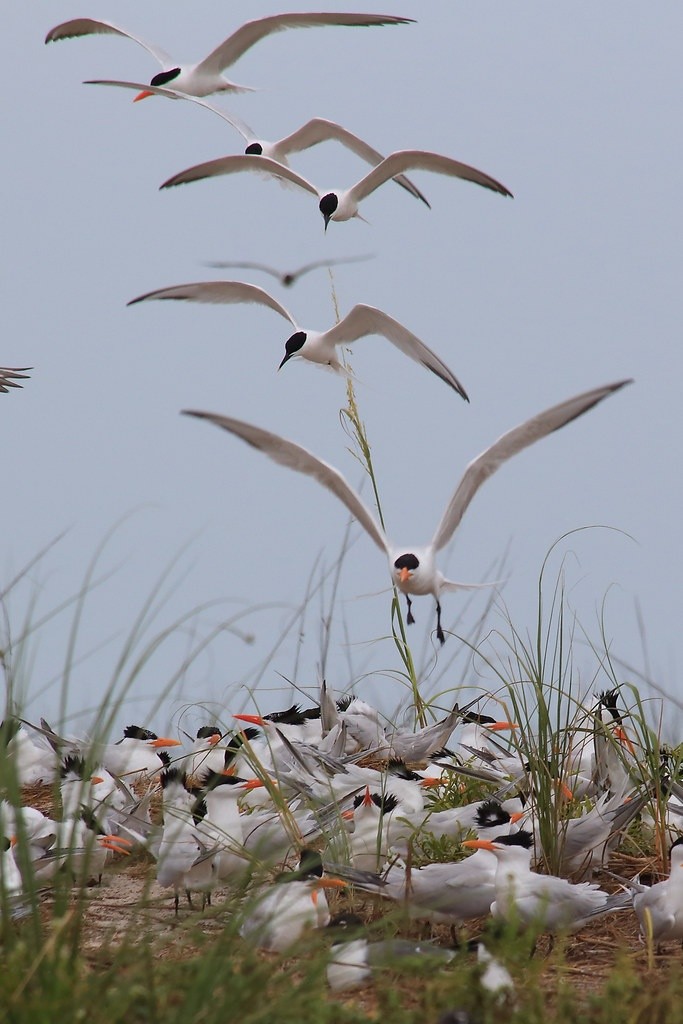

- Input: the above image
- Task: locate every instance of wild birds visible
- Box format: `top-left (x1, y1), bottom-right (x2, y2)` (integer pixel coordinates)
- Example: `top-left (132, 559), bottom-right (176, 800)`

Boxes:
top-left (75, 69), bottom-right (436, 212)
top-left (158, 148), bottom-right (517, 240)
top-left (198, 249), bottom-right (378, 289)
top-left (40, 8), bottom-right (420, 104)
top-left (0, 662), bottom-right (683, 1003)
top-left (177, 370), bottom-right (635, 645)
top-left (123, 276), bottom-right (474, 408)
